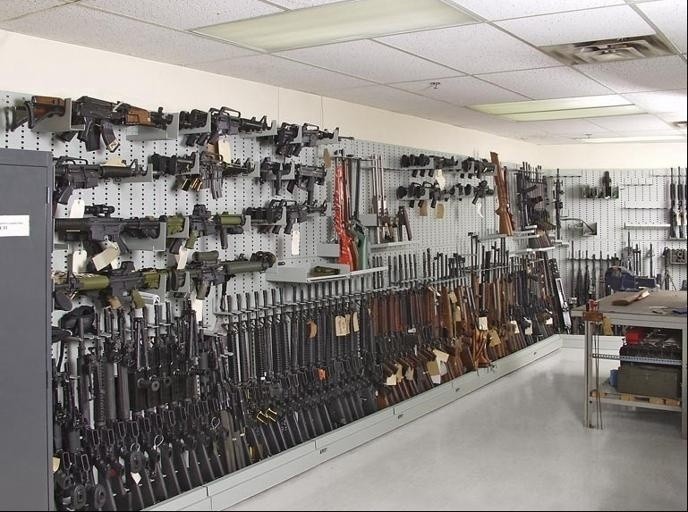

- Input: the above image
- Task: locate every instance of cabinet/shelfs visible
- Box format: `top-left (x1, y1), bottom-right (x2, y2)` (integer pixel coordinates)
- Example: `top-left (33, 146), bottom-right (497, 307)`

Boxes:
top-left (0, 147), bottom-right (58, 512)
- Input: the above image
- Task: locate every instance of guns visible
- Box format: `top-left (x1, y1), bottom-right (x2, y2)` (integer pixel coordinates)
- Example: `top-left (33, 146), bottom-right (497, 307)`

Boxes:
top-left (670, 168), bottom-right (677, 238)
top-left (6, 97), bottom-right (173, 153)
top-left (373, 234), bottom-right (570, 414)
top-left (248, 197), bottom-right (329, 236)
top-left (54, 202), bottom-right (168, 271)
top-left (395, 154), bottom-right (496, 208)
top-left (257, 155), bottom-right (328, 205)
top-left (165, 249), bottom-right (277, 302)
top-left (272, 121), bottom-right (353, 160)
top-left (222, 281), bottom-right (371, 475)
top-left (504, 166), bottom-right (515, 231)
top-left (49, 259), bottom-right (170, 307)
top-left (678, 167), bottom-right (684, 238)
top-left (370, 154), bottom-right (394, 243)
top-left (340, 148), bottom-right (359, 270)
top-left (51, 301), bottom-right (221, 511)
top-left (571, 232), bottom-right (669, 335)
top-left (150, 152), bottom-right (255, 198)
top-left (517, 161), bottom-right (556, 230)
top-left (554, 169), bottom-right (563, 240)
top-left (51, 155), bottom-right (149, 211)
top-left (347, 154), bottom-right (371, 271)
top-left (147, 202), bottom-right (246, 253)
top-left (331, 149), bottom-right (353, 263)
top-left (166, 105), bottom-right (274, 146)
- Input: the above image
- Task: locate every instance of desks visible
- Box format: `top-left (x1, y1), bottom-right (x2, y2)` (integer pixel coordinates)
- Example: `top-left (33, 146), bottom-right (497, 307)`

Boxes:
top-left (569, 290), bottom-right (688, 439)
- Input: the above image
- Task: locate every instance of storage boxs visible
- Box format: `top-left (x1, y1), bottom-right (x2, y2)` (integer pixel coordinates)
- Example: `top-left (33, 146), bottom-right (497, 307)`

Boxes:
top-left (616, 364), bottom-right (682, 399)
